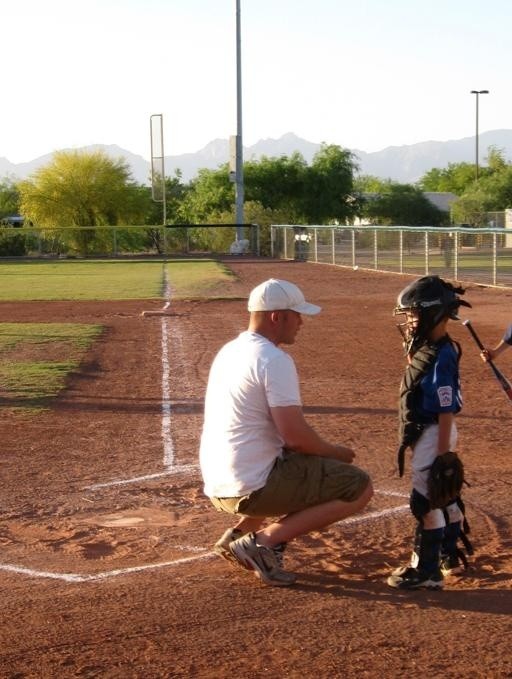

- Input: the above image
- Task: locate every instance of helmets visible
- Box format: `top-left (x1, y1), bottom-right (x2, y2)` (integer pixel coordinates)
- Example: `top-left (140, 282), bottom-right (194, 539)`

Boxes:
top-left (392, 275), bottom-right (459, 353)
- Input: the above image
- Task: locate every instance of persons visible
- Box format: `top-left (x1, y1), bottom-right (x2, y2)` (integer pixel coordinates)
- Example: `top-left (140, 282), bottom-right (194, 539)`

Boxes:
top-left (380, 274), bottom-right (477, 590)
top-left (199, 277), bottom-right (374, 589)
top-left (479, 322), bottom-right (512, 364)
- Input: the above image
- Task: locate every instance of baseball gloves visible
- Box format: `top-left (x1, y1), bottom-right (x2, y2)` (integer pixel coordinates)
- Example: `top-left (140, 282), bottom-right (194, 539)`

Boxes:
top-left (425, 451), bottom-right (461, 508)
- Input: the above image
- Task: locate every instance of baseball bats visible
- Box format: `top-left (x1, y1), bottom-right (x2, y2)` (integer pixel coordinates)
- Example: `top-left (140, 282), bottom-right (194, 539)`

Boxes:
top-left (142, 310), bottom-right (187, 316)
top-left (462, 318), bottom-right (512, 402)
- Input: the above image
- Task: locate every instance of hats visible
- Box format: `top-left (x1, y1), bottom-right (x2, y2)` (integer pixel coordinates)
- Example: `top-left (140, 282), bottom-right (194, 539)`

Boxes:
top-left (245, 275), bottom-right (325, 318)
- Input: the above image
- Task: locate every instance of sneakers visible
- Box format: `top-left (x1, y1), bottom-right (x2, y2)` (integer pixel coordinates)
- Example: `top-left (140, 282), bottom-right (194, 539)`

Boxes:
top-left (228, 531), bottom-right (301, 589)
top-left (387, 564), bottom-right (450, 589)
top-left (439, 552), bottom-right (465, 576)
top-left (210, 525), bottom-right (262, 577)
